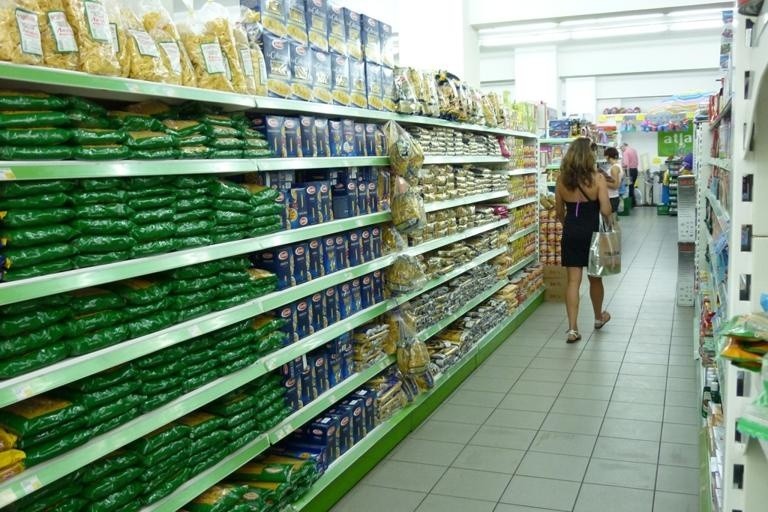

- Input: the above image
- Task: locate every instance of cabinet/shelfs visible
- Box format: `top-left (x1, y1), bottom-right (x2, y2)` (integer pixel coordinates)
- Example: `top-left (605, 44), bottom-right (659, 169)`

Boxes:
top-left (676, 0), bottom-right (768, 512)
top-left (0, 62), bottom-right (545, 512)
top-left (540, 113), bottom-right (623, 302)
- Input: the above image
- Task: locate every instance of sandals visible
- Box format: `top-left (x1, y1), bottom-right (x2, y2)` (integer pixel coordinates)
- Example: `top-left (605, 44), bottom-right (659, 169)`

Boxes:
top-left (595, 311), bottom-right (610, 328)
top-left (567, 329), bottom-right (581, 343)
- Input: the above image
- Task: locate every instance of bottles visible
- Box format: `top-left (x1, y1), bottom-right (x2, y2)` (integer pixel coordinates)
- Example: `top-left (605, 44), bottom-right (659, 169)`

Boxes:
top-left (701, 367), bottom-right (719, 418)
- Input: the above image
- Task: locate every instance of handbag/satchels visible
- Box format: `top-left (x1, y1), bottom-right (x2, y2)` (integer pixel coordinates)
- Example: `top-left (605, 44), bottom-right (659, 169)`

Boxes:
top-left (587, 231), bottom-right (621, 277)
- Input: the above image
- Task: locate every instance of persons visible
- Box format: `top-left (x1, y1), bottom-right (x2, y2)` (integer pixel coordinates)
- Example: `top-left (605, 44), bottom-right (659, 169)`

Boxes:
top-left (620, 142), bottom-right (642, 208)
top-left (604, 148), bottom-right (623, 213)
top-left (555, 137), bottom-right (612, 344)
